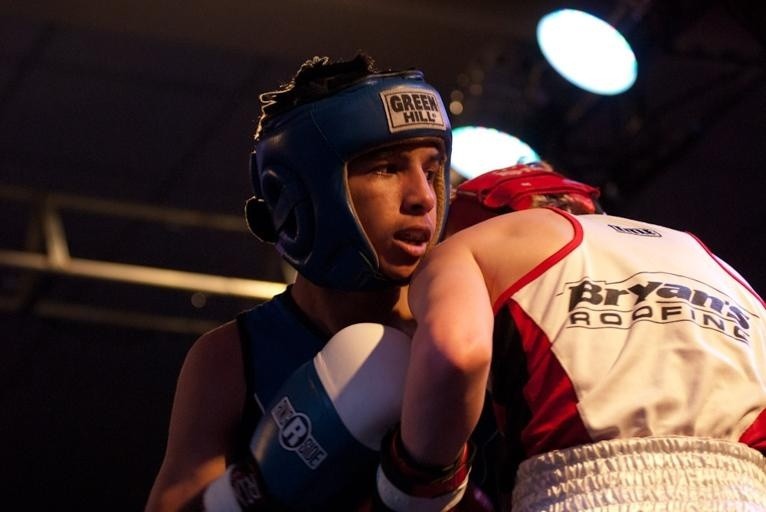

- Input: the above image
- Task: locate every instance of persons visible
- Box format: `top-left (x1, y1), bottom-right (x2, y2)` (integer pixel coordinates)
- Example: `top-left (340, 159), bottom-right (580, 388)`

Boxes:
top-left (144, 48), bottom-right (456, 512)
top-left (364, 125), bottom-right (765, 511)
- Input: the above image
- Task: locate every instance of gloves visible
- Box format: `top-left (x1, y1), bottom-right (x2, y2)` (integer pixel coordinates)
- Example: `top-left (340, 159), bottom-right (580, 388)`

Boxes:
top-left (239, 321), bottom-right (410, 512)
top-left (327, 426), bottom-right (473, 512)
